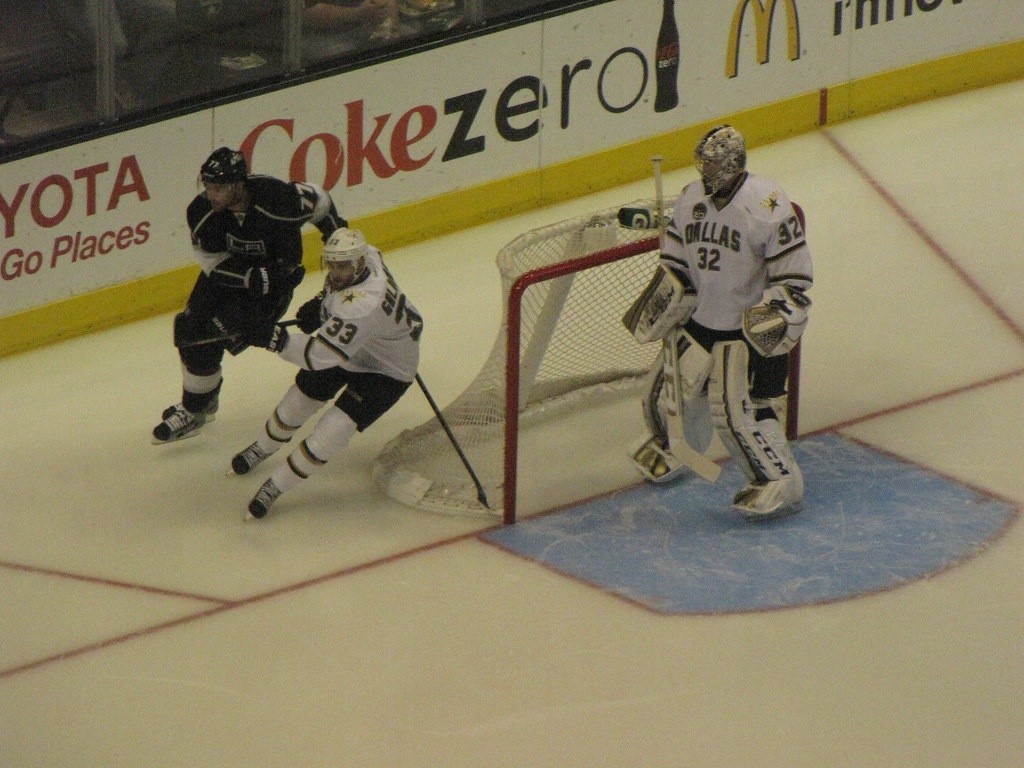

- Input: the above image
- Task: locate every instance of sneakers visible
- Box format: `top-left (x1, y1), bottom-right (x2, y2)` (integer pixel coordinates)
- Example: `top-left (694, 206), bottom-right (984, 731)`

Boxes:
top-left (224, 440), bottom-right (272, 478)
top-left (243, 477), bottom-right (282, 522)
top-left (151, 376), bottom-right (207, 448)
top-left (161, 376), bottom-right (224, 422)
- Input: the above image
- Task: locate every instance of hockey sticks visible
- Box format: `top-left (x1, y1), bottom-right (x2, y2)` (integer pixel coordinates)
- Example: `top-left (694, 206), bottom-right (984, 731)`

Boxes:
top-left (172, 311), bottom-right (304, 350)
top-left (413, 371), bottom-right (490, 512)
top-left (651, 156), bottom-right (722, 485)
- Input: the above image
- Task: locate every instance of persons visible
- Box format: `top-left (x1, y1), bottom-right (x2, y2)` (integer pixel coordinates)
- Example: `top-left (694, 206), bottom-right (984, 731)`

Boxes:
top-left (621, 124), bottom-right (814, 523)
top-left (301, 0), bottom-right (399, 31)
top-left (151, 147), bottom-right (349, 445)
top-left (226, 227), bottom-right (423, 521)
top-left (83, 0), bottom-right (178, 60)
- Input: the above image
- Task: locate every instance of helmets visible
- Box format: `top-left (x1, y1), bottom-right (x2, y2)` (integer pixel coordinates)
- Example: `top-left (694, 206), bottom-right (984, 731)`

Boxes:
top-left (694, 124), bottom-right (747, 195)
top-left (322, 227), bottom-right (367, 278)
top-left (200, 147), bottom-right (247, 193)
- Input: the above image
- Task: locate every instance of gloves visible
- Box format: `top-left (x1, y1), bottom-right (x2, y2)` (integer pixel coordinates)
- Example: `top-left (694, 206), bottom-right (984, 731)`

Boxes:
top-left (319, 214), bottom-right (348, 241)
top-left (296, 297), bottom-right (323, 334)
top-left (241, 315), bottom-right (288, 353)
top-left (254, 264), bottom-right (305, 304)
